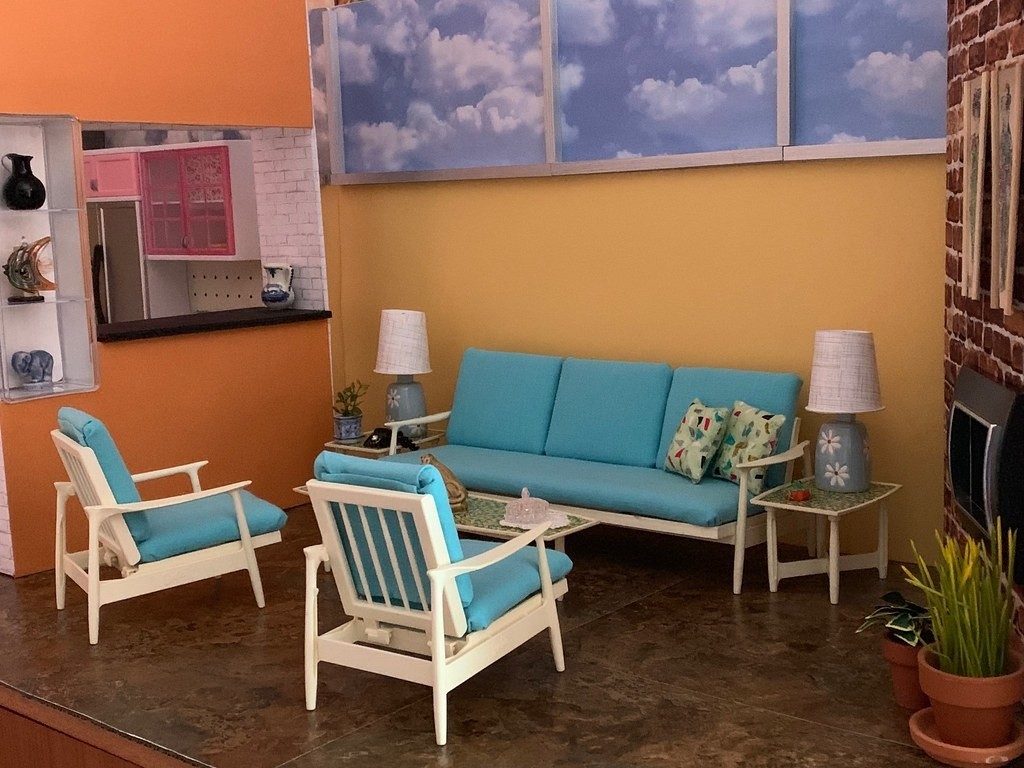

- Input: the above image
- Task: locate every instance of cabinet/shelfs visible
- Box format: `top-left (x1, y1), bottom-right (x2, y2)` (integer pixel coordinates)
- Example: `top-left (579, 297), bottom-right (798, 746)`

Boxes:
top-left (83, 140), bottom-right (261, 323)
top-left (0, 112), bottom-right (101, 405)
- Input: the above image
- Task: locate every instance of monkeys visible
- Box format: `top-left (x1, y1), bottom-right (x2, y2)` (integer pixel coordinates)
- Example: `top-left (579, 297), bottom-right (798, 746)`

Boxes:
top-left (420, 453), bottom-right (468, 506)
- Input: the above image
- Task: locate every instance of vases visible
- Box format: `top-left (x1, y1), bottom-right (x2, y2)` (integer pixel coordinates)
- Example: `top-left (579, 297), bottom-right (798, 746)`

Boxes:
top-left (916, 645), bottom-right (1024, 748)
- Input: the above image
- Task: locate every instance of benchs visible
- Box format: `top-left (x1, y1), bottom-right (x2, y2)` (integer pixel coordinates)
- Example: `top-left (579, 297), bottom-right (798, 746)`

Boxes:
top-left (383, 410), bottom-right (812, 596)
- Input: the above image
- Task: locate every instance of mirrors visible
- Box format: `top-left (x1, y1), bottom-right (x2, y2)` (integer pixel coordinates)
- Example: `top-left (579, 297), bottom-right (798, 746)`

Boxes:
top-left (80, 117), bottom-right (326, 324)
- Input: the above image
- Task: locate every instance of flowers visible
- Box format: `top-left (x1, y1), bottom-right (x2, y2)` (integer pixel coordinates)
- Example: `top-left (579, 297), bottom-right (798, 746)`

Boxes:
top-left (901, 515), bottom-right (1022, 677)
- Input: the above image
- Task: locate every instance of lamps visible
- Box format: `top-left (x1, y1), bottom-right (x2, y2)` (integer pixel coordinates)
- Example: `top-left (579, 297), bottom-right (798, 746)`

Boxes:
top-left (801, 329), bottom-right (887, 492)
top-left (372, 309), bottom-right (433, 439)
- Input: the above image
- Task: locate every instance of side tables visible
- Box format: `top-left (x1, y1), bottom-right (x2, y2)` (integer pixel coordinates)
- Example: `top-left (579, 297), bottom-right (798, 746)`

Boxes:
top-left (325, 428), bottom-right (445, 459)
top-left (750, 475), bottom-right (904, 606)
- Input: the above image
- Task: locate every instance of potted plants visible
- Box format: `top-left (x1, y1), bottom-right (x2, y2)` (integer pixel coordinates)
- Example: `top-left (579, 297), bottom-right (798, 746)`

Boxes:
top-left (331, 379), bottom-right (370, 439)
top-left (855, 589), bottom-right (936, 710)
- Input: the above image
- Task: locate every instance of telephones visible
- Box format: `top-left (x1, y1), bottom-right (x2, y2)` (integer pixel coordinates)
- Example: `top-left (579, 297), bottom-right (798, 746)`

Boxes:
top-left (363, 427), bottom-right (404, 449)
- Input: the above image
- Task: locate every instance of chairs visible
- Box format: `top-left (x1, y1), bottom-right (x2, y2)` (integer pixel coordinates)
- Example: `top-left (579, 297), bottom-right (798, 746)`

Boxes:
top-left (302, 479), bottom-right (568, 747)
top-left (50, 427), bottom-right (282, 647)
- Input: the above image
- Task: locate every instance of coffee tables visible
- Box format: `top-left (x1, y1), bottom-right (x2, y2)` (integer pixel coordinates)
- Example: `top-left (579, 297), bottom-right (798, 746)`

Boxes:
top-left (295, 485), bottom-right (601, 601)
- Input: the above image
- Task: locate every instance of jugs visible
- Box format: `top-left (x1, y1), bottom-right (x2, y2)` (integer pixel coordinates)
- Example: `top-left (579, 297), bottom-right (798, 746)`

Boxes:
top-left (262, 263), bottom-right (294, 309)
top-left (2, 153), bottom-right (45, 211)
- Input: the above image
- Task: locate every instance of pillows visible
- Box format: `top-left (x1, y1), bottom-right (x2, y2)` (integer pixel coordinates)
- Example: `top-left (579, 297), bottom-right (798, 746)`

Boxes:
top-left (709, 400), bottom-right (786, 496)
top-left (663, 398), bottom-right (730, 484)
